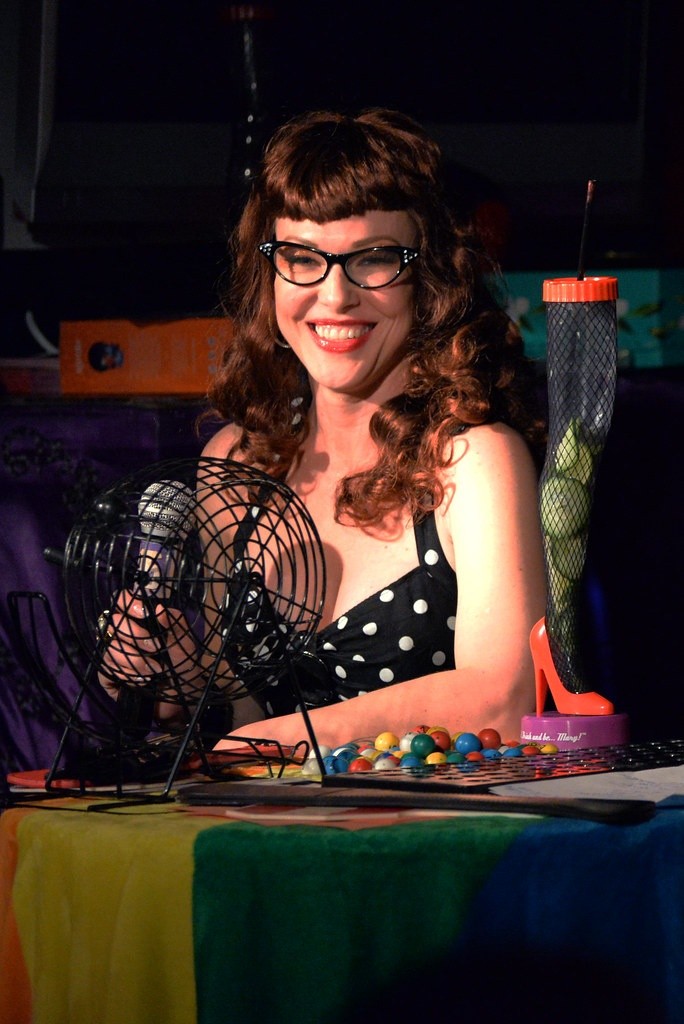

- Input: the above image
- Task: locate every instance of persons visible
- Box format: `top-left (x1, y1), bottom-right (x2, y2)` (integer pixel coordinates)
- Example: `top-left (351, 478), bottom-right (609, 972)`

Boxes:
top-left (98, 109), bottom-right (547, 755)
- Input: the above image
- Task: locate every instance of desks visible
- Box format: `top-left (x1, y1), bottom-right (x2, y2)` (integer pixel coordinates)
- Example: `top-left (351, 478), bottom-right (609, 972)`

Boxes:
top-left (0, 779), bottom-right (683, 1023)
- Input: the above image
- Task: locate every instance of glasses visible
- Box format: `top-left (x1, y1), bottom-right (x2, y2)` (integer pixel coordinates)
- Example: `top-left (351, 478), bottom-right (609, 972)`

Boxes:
top-left (257, 240), bottom-right (418, 289)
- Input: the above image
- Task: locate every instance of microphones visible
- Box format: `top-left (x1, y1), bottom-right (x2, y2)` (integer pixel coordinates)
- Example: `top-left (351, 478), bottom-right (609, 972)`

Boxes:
top-left (111, 480), bottom-right (198, 738)
